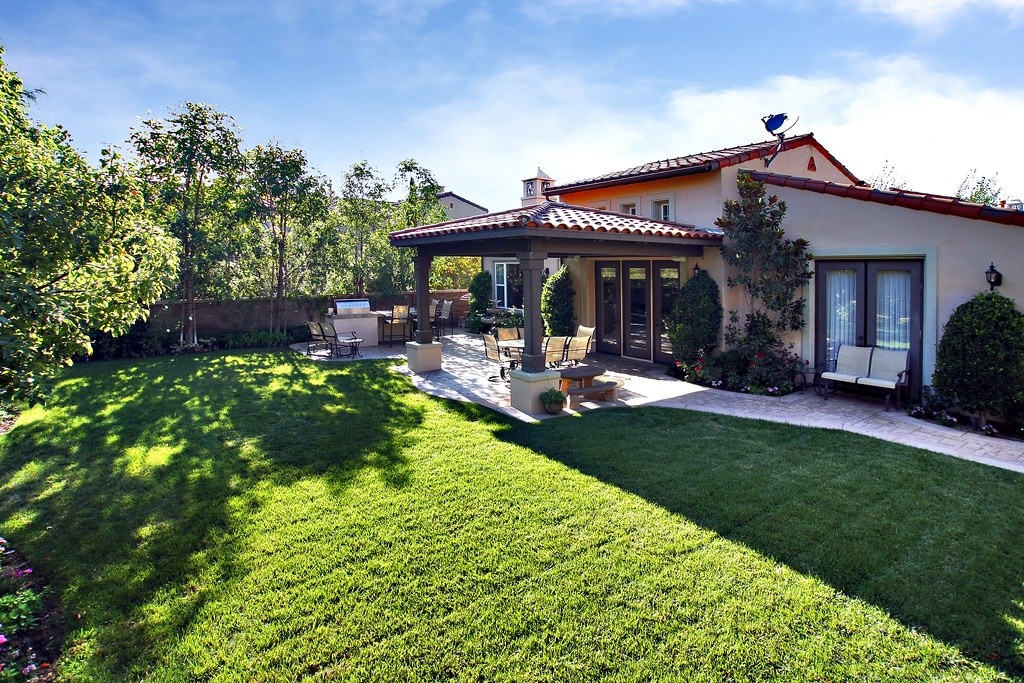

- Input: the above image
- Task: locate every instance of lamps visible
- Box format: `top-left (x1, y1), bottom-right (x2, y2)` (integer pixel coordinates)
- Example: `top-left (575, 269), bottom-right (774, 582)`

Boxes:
top-left (984, 262), bottom-right (1002, 291)
top-left (692, 262), bottom-right (701, 277)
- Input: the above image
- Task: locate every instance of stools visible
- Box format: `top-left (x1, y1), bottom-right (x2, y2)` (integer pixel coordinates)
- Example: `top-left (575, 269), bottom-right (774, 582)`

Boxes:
top-left (458, 316), bottom-right (468, 328)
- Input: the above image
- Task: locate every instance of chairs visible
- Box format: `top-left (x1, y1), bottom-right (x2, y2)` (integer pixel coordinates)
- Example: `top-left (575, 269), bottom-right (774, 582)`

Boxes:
top-left (382, 304), bottom-right (410, 348)
top-left (480, 332), bottom-right (519, 382)
top-left (304, 321), bottom-right (356, 357)
top-left (410, 299), bottom-right (453, 342)
top-left (497, 325), bottom-right (596, 374)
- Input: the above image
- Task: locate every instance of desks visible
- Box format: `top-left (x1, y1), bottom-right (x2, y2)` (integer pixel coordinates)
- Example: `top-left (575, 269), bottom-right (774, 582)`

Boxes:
top-left (344, 339), bottom-right (364, 359)
top-left (793, 368), bottom-right (819, 395)
top-left (376, 307), bottom-right (417, 342)
top-left (496, 336), bottom-right (597, 348)
top-left (553, 366), bottom-right (606, 397)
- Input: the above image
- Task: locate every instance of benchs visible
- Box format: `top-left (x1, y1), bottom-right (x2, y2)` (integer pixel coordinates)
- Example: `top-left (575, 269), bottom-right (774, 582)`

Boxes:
top-left (818, 343), bottom-right (910, 411)
top-left (566, 382), bottom-right (618, 410)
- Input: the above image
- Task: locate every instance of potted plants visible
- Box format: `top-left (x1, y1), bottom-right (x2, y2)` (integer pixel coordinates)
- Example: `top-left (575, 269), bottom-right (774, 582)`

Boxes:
top-left (539, 387), bottom-right (566, 415)
